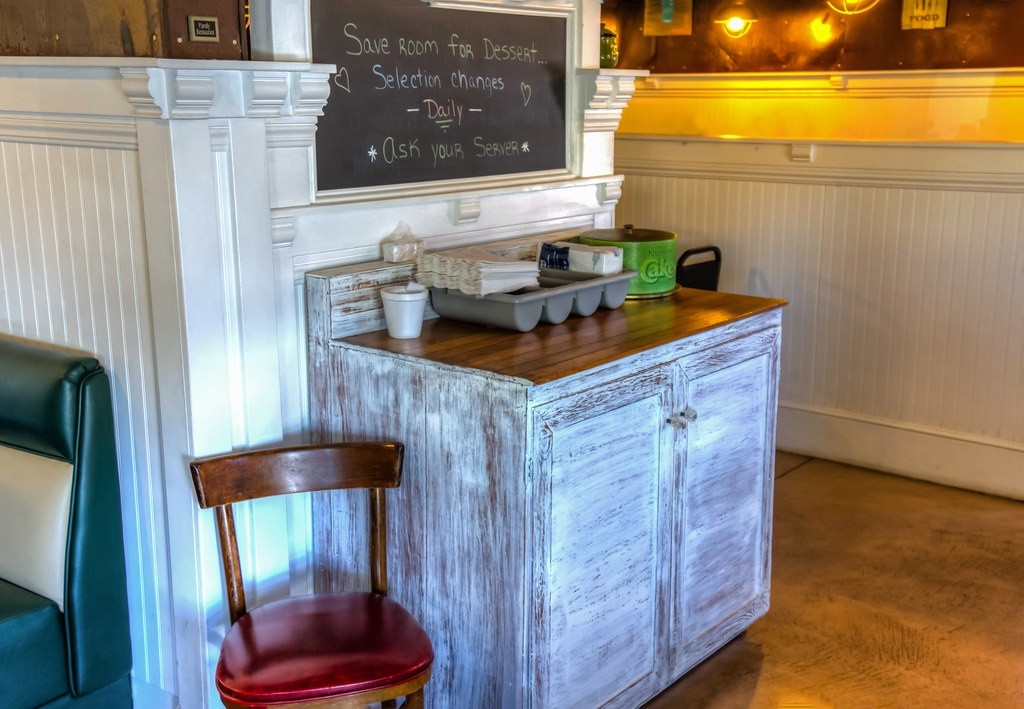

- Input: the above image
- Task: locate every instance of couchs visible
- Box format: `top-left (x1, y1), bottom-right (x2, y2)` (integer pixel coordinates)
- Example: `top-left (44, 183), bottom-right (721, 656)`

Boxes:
top-left (0, 335), bottom-right (134, 709)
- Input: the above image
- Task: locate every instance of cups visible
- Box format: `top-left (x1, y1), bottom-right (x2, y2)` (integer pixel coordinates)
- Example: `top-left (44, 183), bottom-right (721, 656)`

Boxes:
top-left (380, 286), bottom-right (428, 338)
top-left (600, 23), bottom-right (619, 69)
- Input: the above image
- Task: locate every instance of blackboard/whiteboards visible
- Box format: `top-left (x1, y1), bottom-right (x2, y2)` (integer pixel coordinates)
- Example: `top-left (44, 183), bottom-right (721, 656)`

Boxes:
top-left (305, 0), bottom-right (578, 206)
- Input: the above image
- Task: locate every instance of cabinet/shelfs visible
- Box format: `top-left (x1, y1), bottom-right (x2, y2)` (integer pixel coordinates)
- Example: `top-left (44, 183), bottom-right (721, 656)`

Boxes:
top-left (304, 225), bottom-right (791, 709)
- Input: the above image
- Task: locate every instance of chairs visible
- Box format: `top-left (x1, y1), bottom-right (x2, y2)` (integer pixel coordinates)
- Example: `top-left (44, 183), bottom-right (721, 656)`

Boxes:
top-left (189, 442), bottom-right (436, 709)
